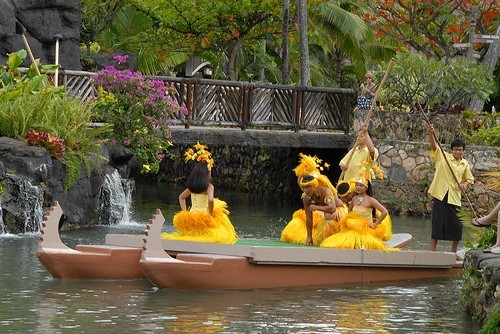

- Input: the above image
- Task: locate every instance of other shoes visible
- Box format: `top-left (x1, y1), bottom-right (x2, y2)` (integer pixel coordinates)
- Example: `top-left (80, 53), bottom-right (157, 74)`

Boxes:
top-left (490, 246), bottom-right (500, 253)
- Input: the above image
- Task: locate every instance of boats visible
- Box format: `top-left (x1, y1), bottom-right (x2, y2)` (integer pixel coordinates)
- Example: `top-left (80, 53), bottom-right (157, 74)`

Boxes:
top-left (37, 199), bottom-right (465, 289)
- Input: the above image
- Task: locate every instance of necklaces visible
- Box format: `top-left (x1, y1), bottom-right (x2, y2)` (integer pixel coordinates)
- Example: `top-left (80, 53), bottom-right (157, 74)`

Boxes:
top-left (358, 195), bottom-right (367, 205)
top-left (311, 194), bottom-right (320, 201)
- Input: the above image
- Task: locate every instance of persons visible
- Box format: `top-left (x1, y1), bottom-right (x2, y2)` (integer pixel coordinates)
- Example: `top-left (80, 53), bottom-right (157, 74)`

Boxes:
top-left (358, 72), bottom-right (376, 110)
top-left (161, 155), bottom-right (240, 244)
top-left (470, 201), bottom-right (500, 253)
top-left (339, 123), bottom-right (379, 192)
top-left (320, 176), bottom-right (401, 251)
top-left (280, 174), bottom-right (349, 246)
top-left (428, 125), bottom-right (474, 260)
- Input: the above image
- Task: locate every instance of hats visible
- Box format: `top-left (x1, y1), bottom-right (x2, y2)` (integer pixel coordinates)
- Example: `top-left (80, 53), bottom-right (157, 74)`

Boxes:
top-left (354, 177), bottom-right (367, 188)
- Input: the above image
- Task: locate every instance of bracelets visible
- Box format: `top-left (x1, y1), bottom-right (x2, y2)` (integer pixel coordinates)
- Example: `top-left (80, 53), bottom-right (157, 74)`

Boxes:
top-left (374, 223), bottom-right (377, 226)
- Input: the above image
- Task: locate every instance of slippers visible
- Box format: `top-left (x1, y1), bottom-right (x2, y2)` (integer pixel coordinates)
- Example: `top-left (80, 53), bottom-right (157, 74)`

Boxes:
top-left (483, 246), bottom-right (492, 253)
top-left (471, 217), bottom-right (492, 227)
